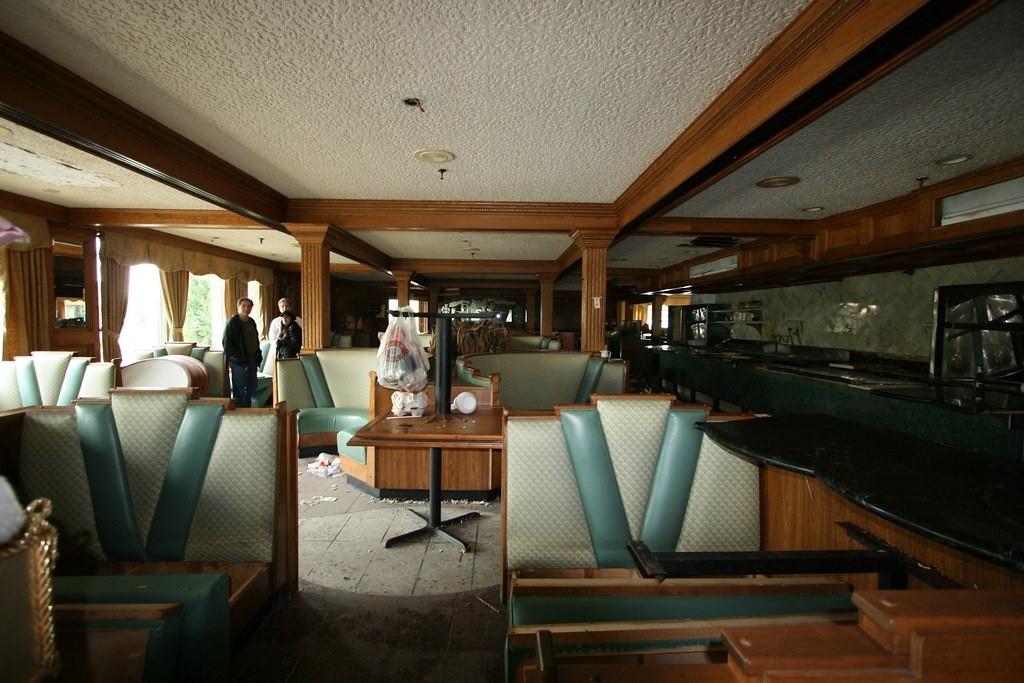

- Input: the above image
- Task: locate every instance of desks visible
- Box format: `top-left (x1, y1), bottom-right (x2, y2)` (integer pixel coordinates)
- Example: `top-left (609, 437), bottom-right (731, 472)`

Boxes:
top-left (345, 401), bottom-right (511, 553)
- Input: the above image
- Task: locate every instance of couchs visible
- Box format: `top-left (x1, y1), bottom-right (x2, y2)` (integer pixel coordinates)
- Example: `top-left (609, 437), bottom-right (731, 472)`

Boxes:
top-left (0, 328), bottom-right (864, 682)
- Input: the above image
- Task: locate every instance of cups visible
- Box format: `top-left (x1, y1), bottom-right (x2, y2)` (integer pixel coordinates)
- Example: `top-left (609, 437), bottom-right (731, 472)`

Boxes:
top-left (453, 391), bottom-right (479, 415)
top-left (600, 350), bottom-right (611, 358)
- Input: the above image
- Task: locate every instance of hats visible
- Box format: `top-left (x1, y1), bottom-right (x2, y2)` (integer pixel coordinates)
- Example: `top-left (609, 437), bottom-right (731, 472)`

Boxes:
top-left (280, 310), bottom-right (296, 320)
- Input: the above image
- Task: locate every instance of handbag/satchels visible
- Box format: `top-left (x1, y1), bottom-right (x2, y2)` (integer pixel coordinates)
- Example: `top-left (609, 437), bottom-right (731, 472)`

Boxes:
top-left (277, 343), bottom-right (292, 358)
top-left (375, 309), bottom-right (428, 392)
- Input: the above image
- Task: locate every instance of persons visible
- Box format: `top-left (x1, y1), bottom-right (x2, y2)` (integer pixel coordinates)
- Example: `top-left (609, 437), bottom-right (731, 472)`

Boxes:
top-left (222, 297), bottom-right (263, 407)
top-left (269, 298), bottom-right (302, 360)
